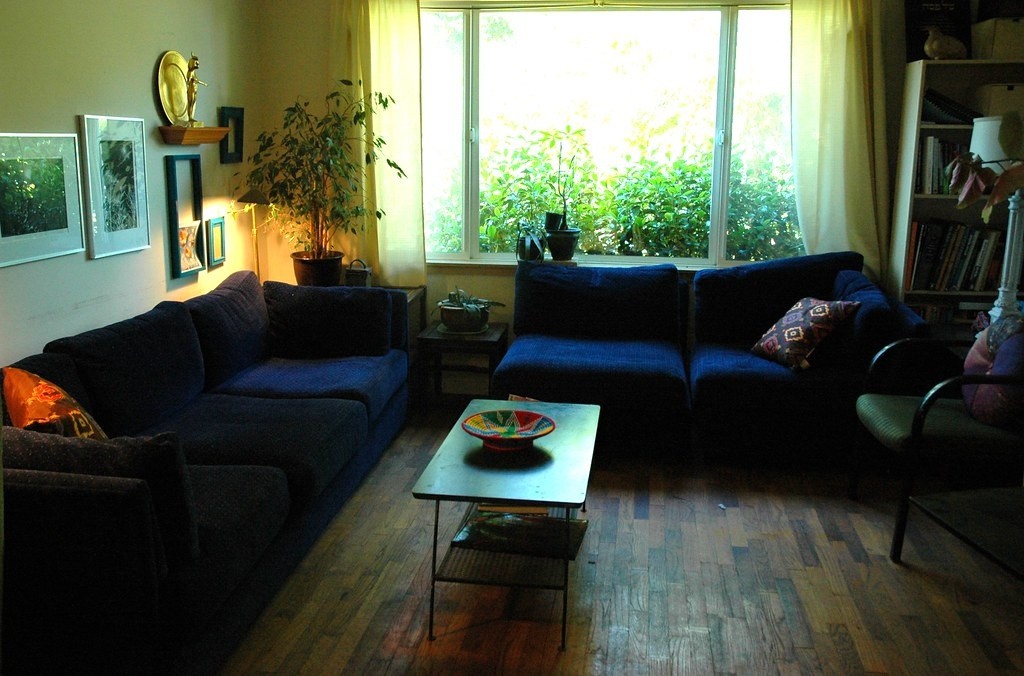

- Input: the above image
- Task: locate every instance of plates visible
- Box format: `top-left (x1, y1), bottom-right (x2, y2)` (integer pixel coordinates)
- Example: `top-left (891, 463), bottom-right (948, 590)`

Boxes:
top-left (461, 409), bottom-right (556, 452)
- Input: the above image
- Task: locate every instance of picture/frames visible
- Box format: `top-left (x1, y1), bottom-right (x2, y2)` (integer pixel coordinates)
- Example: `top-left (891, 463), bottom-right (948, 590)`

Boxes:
top-left (164, 154), bottom-right (207, 279)
top-left (220, 106), bottom-right (243, 164)
top-left (79, 115), bottom-right (150, 260)
top-left (0, 132), bottom-right (87, 268)
top-left (209, 216), bottom-right (226, 267)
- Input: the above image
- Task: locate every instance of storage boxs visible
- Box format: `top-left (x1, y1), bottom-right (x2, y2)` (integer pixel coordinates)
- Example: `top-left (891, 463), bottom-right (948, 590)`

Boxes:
top-left (973, 84), bottom-right (1024, 120)
top-left (970, 17), bottom-right (1024, 59)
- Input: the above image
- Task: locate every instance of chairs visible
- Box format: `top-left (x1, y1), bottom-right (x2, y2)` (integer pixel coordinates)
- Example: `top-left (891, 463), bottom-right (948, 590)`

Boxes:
top-left (846, 316), bottom-right (1024, 562)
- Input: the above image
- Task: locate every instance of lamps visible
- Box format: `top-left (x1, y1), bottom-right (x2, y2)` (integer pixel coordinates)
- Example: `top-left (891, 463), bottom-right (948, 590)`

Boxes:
top-left (969, 114), bottom-right (1024, 326)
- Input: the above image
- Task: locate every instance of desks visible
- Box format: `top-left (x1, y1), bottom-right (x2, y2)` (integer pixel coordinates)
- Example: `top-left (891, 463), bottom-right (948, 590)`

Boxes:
top-left (411, 399), bottom-right (600, 652)
top-left (378, 284), bottom-right (427, 408)
top-left (416, 319), bottom-right (509, 417)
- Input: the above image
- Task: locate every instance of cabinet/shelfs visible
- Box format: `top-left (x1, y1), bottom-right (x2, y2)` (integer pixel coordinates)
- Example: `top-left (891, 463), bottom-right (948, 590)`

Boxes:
top-left (883, 59), bottom-right (1024, 363)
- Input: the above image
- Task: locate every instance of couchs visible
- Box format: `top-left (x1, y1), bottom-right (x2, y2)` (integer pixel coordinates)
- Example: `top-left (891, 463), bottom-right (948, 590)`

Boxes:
top-left (0, 270), bottom-right (416, 676)
top-left (489, 250), bottom-right (929, 473)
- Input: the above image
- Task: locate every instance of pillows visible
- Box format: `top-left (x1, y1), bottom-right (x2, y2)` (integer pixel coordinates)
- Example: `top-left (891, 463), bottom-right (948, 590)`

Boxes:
top-left (3, 365), bottom-right (107, 440)
top-left (961, 314), bottom-right (1024, 425)
top-left (750, 296), bottom-right (862, 373)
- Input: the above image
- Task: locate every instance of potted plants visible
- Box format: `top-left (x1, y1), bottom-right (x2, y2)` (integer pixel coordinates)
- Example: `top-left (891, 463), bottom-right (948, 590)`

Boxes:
top-left (545, 140), bottom-right (581, 260)
top-left (514, 220), bottom-right (547, 262)
top-left (438, 284), bottom-right (507, 332)
top-left (225, 78), bottom-right (409, 287)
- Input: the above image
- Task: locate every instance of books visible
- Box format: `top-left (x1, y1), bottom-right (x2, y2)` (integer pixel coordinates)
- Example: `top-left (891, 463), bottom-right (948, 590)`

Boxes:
top-left (914, 137), bottom-right (968, 196)
top-left (904, 222), bottom-right (1002, 293)
top-left (906, 304), bottom-right (953, 338)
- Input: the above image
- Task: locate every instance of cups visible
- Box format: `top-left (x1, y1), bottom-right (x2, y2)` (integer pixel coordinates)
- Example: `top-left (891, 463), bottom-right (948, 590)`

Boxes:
top-left (544, 211), bottom-right (563, 231)
